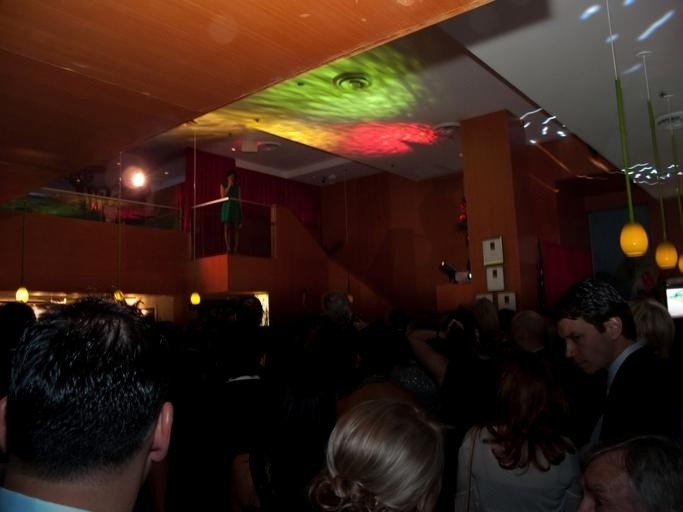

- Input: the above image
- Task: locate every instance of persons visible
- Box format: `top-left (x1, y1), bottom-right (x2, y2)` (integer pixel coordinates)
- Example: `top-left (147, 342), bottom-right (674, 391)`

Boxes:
top-left (1, 278), bottom-right (683, 511)
top-left (220, 170), bottom-right (241, 254)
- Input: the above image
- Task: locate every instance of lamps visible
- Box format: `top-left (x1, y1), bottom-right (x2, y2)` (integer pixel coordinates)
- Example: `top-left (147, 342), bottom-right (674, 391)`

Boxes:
top-left (607, 0), bottom-right (683, 273)
top-left (8, 114), bottom-right (206, 312)
